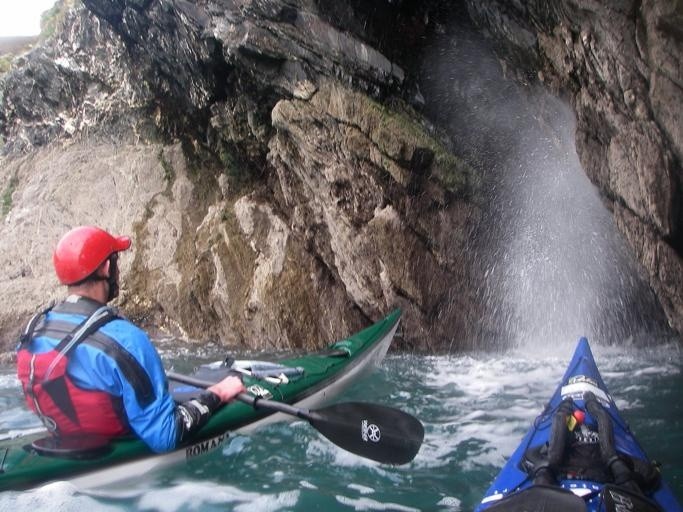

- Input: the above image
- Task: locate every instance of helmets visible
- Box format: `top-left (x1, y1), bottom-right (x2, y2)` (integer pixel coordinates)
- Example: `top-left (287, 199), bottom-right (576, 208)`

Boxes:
top-left (53, 224), bottom-right (131, 287)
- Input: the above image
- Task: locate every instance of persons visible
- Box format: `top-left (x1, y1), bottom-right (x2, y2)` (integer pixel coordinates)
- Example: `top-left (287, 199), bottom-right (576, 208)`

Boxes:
top-left (13, 225), bottom-right (248, 454)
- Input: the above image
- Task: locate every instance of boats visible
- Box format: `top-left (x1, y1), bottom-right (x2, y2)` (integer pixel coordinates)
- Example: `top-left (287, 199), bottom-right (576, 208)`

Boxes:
top-left (475, 337), bottom-right (683, 512)
top-left (0, 307), bottom-right (406, 503)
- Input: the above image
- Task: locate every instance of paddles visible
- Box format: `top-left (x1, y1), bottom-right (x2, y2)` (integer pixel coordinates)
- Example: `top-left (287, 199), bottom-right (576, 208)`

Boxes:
top-left (480, 390), bottom-right (659, 511)
top-left (163, 371), bottom-right (423, 465)
